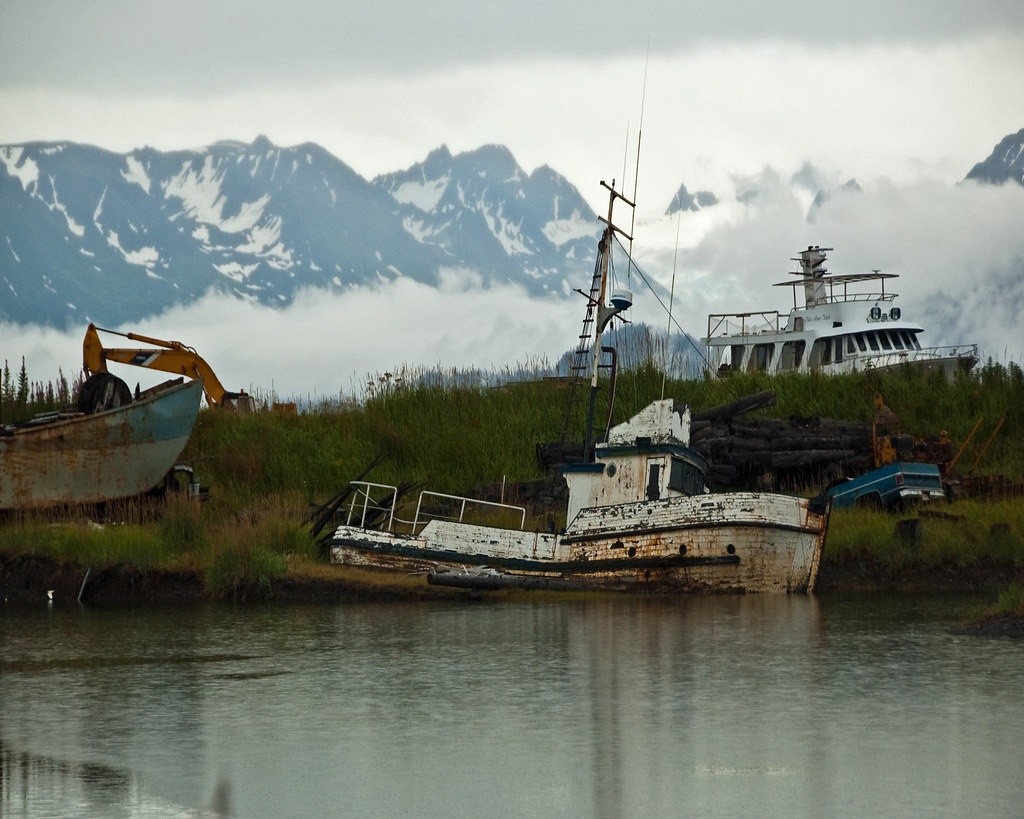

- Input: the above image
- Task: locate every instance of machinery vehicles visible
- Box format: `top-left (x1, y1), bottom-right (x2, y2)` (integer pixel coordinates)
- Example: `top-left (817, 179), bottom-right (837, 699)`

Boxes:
top-left (80, 323), bottom-right (298, 420)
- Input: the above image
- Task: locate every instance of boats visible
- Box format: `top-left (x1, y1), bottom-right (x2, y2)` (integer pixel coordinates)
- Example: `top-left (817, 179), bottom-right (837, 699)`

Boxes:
top-left (266, 174), bottom-right (834, 602)
top-left (0, 370), bottom-right (211, 516)
top-left (702, 244), bottom-right (980, 382)
